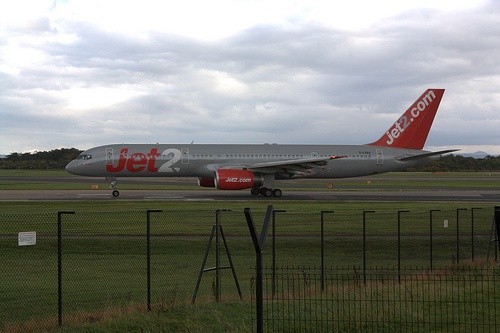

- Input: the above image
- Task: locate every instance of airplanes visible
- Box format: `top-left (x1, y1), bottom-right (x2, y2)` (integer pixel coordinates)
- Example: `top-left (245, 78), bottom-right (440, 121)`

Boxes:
top-left (65, 89), bottom-right (462, 198)
